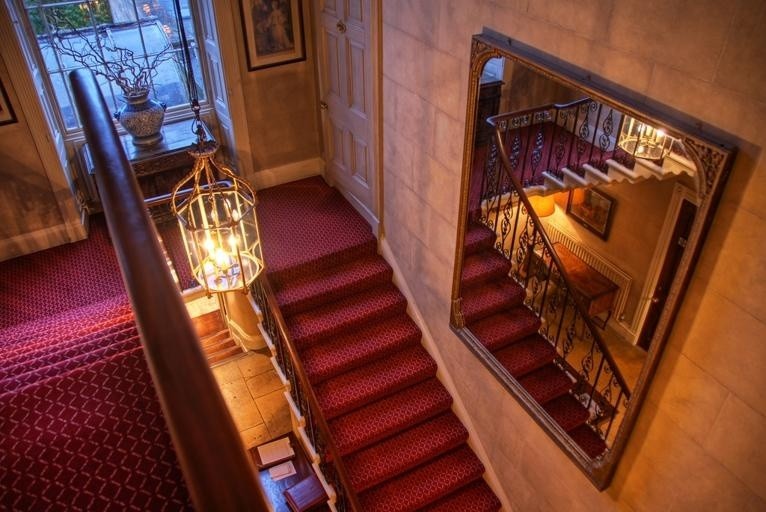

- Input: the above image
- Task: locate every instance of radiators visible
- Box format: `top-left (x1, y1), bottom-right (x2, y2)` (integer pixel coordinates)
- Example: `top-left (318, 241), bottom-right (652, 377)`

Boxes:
top-left (545, 221), bottom-right (634, 321)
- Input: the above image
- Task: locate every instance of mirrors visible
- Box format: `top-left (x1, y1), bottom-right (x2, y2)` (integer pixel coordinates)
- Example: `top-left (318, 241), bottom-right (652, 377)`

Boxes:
top-left (446, 30), bottom-right (742, 493)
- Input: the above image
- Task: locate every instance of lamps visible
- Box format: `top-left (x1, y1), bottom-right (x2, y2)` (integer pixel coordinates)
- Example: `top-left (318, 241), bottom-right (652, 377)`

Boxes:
top-left (617, 114), bottom-right (675, 162)
top-left (166, 1), bottom-right (268, 302)
top-left (527, 194), bottom-right (556, 219)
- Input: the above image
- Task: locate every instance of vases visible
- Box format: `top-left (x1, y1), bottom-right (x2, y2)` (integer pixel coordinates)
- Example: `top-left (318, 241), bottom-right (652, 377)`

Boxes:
top-left (113, 88), bottom-right (168, 148)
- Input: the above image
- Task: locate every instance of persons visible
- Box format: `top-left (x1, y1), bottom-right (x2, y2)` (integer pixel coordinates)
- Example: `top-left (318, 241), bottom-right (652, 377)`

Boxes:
top-left (251, 0), bottom-right (293, 54)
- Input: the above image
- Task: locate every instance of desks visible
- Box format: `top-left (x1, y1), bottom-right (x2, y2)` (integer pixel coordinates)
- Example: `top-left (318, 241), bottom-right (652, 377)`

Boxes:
top-left (532, 241), bottom-right (621, 343)
top-left (82, 117), bottom-right (221, 227)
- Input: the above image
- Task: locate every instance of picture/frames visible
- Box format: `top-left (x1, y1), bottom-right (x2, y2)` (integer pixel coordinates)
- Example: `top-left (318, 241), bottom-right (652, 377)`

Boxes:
top-left (238, 0), bottom-right (307, 74)
top-left (566, 186), bottom-right (620, 242)
top-left (0, 76), bottom-right (19, 127)
